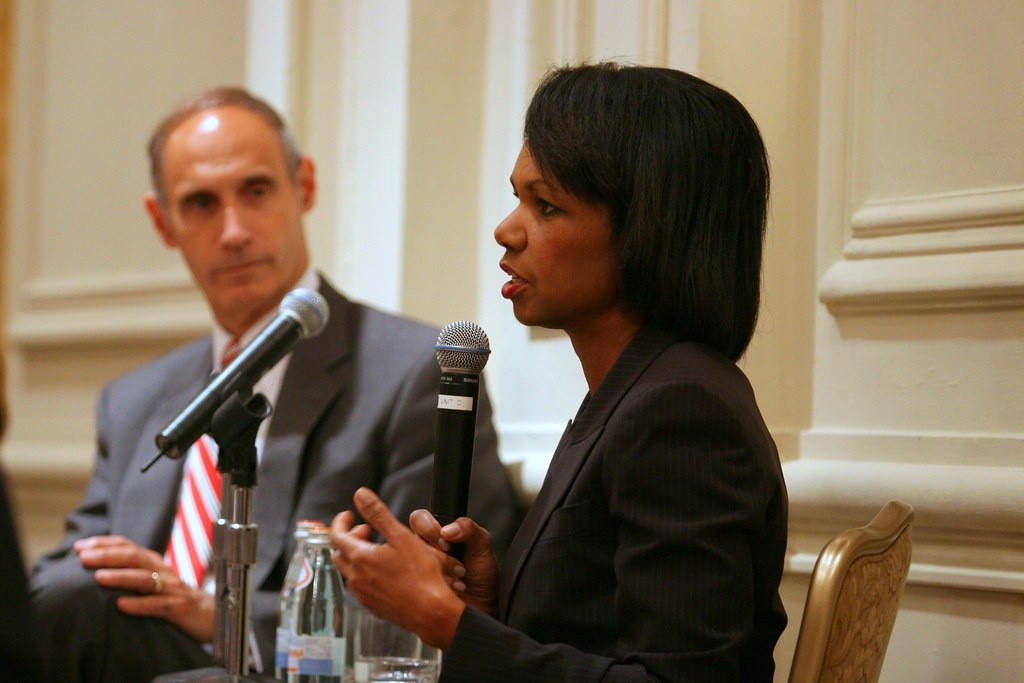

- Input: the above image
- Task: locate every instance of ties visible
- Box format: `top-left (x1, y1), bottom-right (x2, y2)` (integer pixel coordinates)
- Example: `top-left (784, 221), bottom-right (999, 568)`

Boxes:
top-left (163, 337), bottom-right (244, 590)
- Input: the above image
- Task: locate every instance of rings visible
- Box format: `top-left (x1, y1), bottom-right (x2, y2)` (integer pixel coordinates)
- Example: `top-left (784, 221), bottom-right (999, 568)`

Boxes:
top-left (152, 572), bottom-right (163, 592)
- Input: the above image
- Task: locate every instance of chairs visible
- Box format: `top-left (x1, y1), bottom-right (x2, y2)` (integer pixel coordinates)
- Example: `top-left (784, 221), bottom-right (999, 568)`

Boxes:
top-left (788, 499), bottom-right (915, 683)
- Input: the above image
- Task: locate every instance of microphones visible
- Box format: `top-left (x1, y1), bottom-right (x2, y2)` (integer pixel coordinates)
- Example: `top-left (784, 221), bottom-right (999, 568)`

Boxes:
top-left (428, 319), bottom-right (490, 562)
top-left (139, 287), bottom-right (329, 474)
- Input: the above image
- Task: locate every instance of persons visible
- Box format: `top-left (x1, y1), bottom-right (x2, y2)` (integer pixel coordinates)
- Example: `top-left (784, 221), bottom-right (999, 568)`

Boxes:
top-left (31, 86), bottom-right (527, 683)
top-left (327, 61), bottom-right (789, 683)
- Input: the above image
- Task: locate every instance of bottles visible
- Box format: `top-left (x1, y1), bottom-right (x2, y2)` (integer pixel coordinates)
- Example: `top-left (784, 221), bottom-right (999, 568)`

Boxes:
top-left (287, 527), bottom-right (348, 683)
top-left (274, 520), bottom-right (326, 680)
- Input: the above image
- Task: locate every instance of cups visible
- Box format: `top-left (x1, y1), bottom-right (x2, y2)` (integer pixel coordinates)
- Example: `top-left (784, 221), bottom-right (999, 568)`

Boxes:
top-left (353, 608), bottom-right (442, 683)
